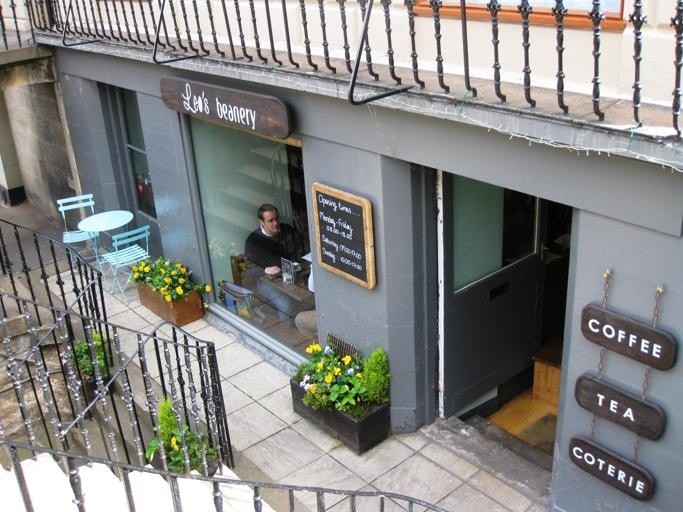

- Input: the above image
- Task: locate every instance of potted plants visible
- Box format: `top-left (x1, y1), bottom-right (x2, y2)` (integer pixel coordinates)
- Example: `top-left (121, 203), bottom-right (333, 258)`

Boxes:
top-left (74, 329), bottom-right (113, 401)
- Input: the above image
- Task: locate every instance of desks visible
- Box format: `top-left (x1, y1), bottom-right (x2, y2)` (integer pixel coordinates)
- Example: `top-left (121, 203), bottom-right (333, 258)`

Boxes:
top-left (78, 210), bottom-right (134, 287)
top-left (258, 274), bottom-right (314, 302)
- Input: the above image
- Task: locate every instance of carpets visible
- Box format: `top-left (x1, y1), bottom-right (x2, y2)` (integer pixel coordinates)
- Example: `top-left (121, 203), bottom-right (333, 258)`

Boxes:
top-left (518, 413), bottom-right (556, 456)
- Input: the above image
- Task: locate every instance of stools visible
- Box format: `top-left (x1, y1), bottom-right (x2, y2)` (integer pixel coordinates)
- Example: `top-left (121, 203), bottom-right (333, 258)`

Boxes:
top-left (531, 338), bottom-right (562, 410)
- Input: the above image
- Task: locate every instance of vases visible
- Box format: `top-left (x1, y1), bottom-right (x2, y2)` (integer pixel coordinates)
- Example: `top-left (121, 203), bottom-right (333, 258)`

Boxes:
top-left (150, 449), bottom-right (175, 480)
top-left (290, 377), bottom-right (388, 456)
top-left (136, 283), bottom-right (204, 328)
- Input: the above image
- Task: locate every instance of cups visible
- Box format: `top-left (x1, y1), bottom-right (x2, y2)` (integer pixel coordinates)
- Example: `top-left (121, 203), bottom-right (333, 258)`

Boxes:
top-left (294, 262), bottom-right (301, 271)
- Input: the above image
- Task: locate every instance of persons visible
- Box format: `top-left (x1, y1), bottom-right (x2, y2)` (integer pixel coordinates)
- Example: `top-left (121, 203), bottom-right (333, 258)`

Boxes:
top-left (244, 203), bottom-right (304, 347)
top-left (293, 263), bottom-right (318, 344)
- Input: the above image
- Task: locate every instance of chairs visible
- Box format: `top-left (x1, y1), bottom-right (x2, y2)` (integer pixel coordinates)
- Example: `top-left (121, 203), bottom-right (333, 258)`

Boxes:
top-left (231, 254), bottom-right (279, 320)
top-left (57, 193), bottom-right (100, 274)
top-left (101, 225), bottom-right (151, 305)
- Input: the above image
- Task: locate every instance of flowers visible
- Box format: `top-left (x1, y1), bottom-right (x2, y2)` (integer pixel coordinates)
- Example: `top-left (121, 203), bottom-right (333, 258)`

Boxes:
top-left (146, 393), bottom-right (217, 475)
top-left (127, 256), bottom-right (212, 309)
top-left (294, 341), bottom-right (392, 418)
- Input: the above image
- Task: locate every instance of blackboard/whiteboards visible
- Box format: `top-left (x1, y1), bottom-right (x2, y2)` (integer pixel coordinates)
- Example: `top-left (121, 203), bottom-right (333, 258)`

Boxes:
top-left (311, 182), bottom-right (376, 290)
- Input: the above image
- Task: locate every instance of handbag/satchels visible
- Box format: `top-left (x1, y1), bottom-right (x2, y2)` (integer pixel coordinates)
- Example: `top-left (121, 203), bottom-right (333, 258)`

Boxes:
top-left (217, 280), bottom-right (266, 323)
